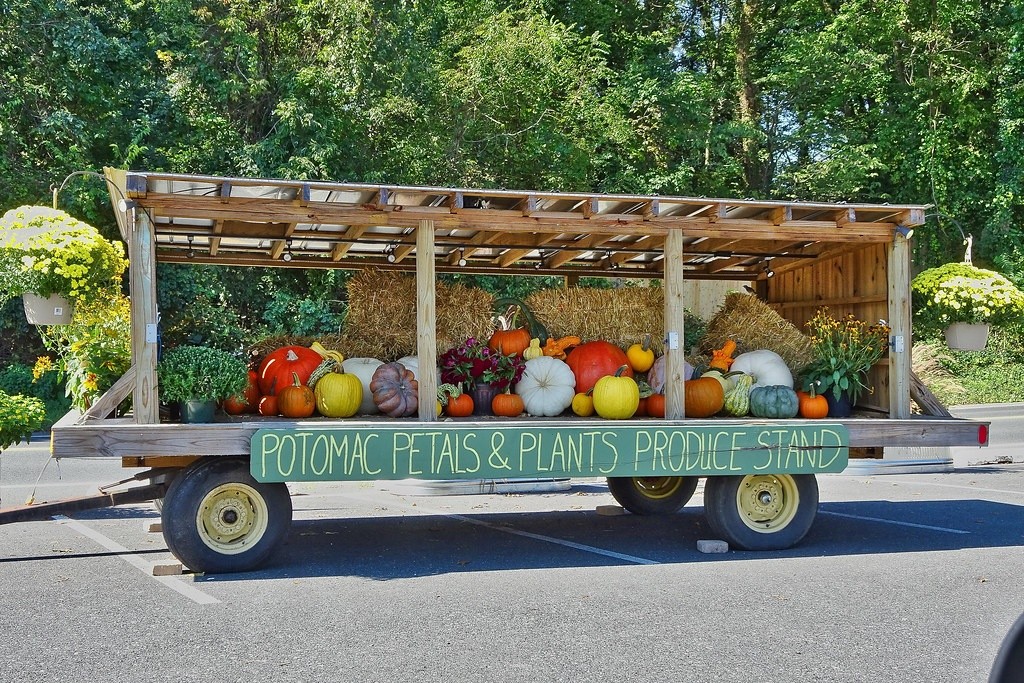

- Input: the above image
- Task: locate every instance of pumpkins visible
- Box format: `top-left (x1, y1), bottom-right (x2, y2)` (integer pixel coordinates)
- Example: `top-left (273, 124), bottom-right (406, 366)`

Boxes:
top-left (646, 340), bottom-right (829, 418)
top-left (432, 298), bottom-right (657, 420)
top-left (220, 342), bottom-right (419, 417)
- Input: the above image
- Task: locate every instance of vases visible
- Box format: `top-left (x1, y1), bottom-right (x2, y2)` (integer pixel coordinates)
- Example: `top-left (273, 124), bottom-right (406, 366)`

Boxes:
top-left (821, 387), bottom-right (850, 418)
top-left (471, 383), bottom-right (502, 416)
top-left (945, 322), bottom-right (989, 350)
top-left (23, 293), bottom-right (74, 325)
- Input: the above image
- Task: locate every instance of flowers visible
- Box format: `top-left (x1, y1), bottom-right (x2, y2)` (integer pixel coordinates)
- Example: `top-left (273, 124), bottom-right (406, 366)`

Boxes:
top-left (440, 336), bottom-right (526, 394)
top-left (0, 204), bottom-right (132, 410)
top-left (799, 305), bottom-right (891, 405)
top-left (911, 262), bottom-right (1024, 325)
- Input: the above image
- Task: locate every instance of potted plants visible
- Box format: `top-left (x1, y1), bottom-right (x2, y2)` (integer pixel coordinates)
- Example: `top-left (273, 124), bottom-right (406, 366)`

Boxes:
top-left (157, 344), bottom-right (249, 423)
